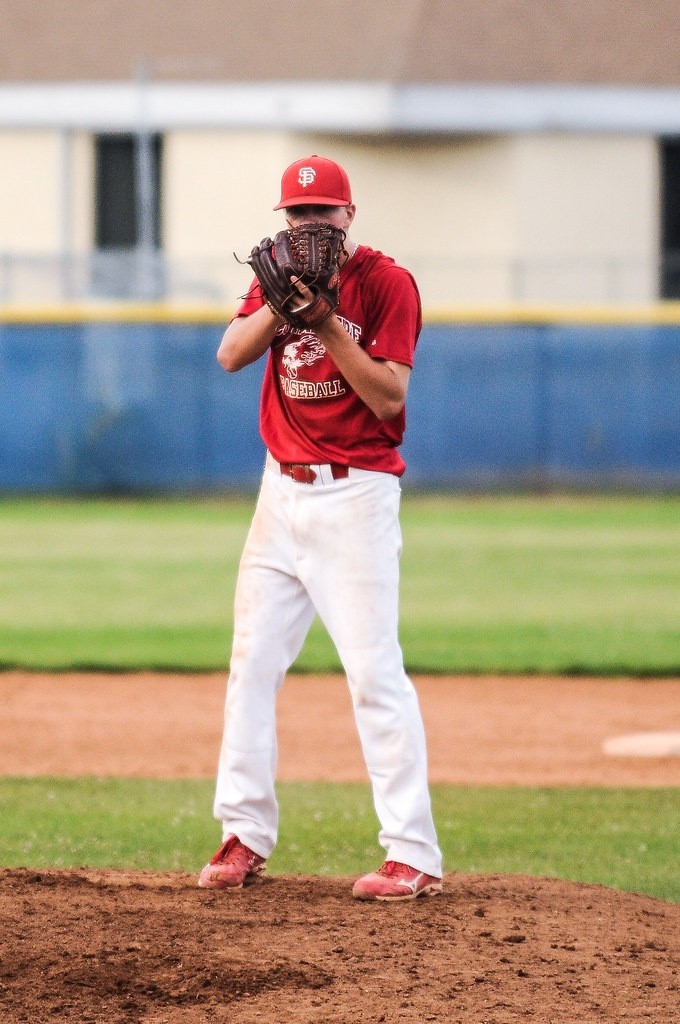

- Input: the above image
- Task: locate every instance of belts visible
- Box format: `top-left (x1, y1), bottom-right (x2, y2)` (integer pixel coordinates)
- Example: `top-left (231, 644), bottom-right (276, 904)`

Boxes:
top-left (280, 459), bottom-right (348, 484)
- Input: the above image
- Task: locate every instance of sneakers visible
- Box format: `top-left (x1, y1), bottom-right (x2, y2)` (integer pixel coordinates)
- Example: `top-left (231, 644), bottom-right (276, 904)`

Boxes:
top-left (198, 833), bottom-right (266, 889)
top-left (353, 862), bottom-right (445, 901)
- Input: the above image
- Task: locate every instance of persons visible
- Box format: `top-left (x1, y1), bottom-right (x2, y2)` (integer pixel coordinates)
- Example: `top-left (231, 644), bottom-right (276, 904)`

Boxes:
top-left (200, 154), bottom-right (445, 901)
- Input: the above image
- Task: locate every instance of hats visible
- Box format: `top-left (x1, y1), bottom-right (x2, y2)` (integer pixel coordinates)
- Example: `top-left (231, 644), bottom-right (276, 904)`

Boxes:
top-left (273, 155), bottom-right (352, 211)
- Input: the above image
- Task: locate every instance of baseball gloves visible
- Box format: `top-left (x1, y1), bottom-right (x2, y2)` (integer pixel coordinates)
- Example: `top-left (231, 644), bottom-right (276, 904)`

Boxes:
top-left (247, 222), bottom-right (345, 331)
